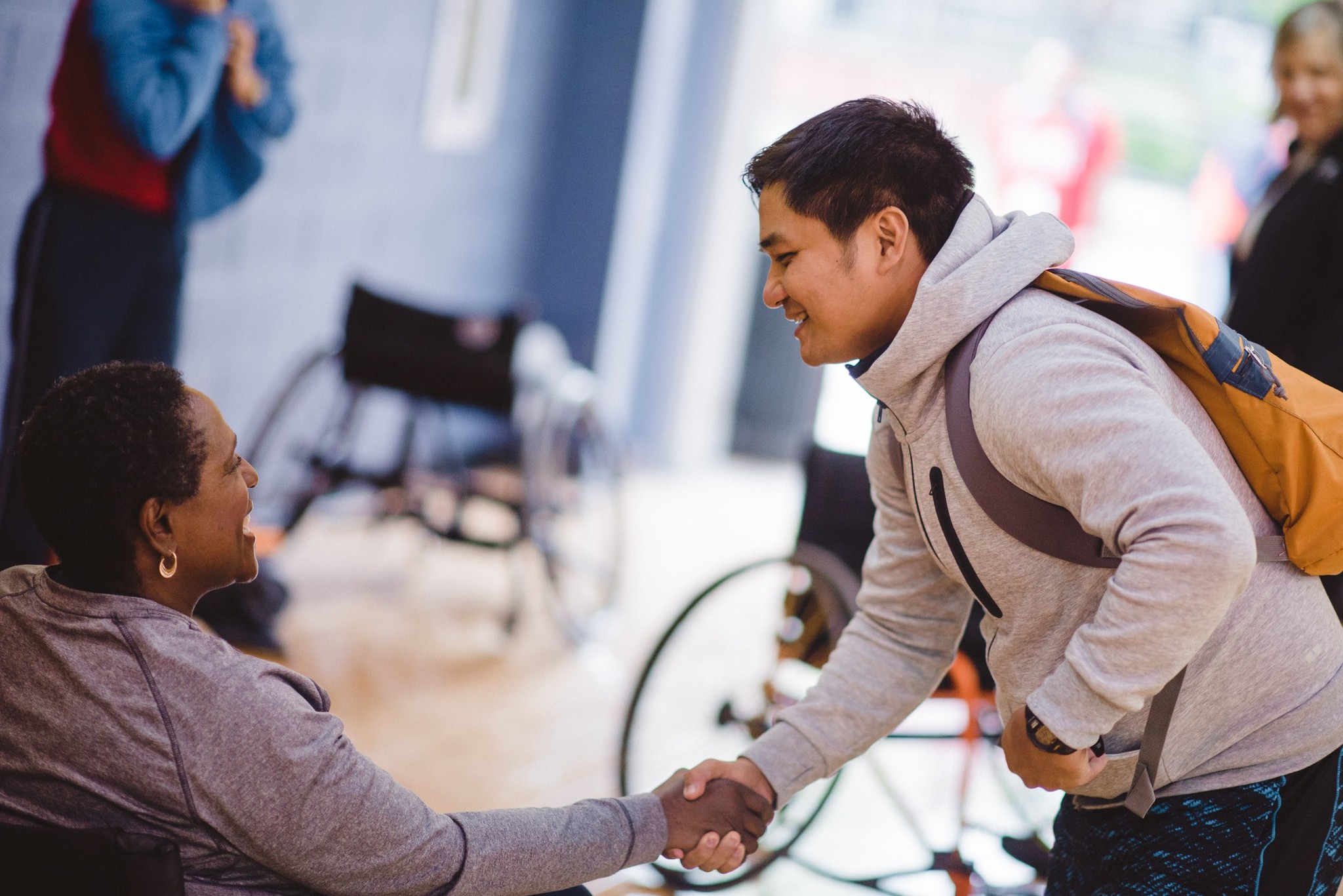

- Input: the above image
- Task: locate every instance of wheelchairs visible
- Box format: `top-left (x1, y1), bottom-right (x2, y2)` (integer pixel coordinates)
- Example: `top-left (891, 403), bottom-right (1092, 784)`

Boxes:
top-left (620, 438), bottom-right (1060, 896)
top-left (239, 282), bottom-right (629, 640)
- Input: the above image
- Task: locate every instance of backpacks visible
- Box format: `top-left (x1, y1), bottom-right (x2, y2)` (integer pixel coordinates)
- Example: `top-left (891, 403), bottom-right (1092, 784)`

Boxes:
top-left (887, 268), bottom-right (1343, 576)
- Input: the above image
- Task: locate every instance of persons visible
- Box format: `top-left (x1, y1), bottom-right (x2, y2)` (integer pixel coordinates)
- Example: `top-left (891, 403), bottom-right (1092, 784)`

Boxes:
top-left (1224, 1), bottom-right (1343, 390)
top-left (0, 365), bottom-right (775, 896)
top-left (0, 0), bottom-right (298, 574)
top-left (662, 96), bottom-right (1343, 896)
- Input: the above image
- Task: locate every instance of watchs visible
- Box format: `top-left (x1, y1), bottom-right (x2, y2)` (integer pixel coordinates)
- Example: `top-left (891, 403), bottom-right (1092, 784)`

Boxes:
top-left (1024, 704), bottom-right (1104, 759)
